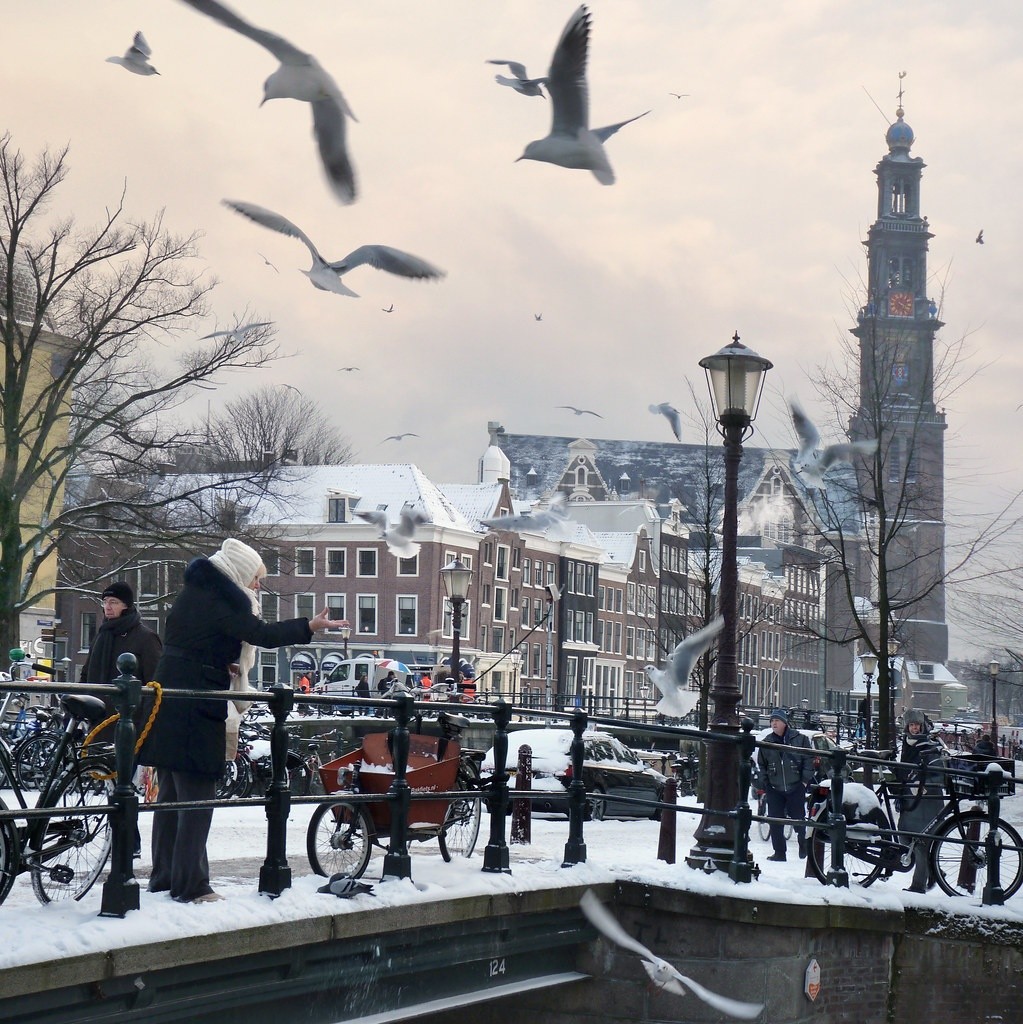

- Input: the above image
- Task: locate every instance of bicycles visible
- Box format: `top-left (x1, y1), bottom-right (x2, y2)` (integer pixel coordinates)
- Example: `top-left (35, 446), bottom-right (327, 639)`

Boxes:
top-left (307, 711), bottom-right (485, 880)
top-left (758, 800), bottom-right (793, 840)
top-left (0, 649), bottom-right (123, 905)
top-left (0, 689), bottom-right (113, 795)
top-left (671, 750), bottom-right (699, 796)
top-left (808, 729), bottom-right (1023, 903)
top-left (215, 705), bottom-right (337, 800)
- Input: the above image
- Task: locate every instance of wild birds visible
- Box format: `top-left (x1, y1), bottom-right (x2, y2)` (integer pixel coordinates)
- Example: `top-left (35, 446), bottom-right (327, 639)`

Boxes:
top-left (558, 406), bottom-right (606, 420)
top-left (487, 59), bottom-right (550, 100)
top-left (649, 401), bottom-right (685, 443)
top-left (514, 4), bottom-right (652, 185)
top-left (976, 229), bottom-right (985, 245)
top-left (337, 367), bottom-right (360, 372)
top-left (668, 92), bottom-right (690, 99)
top-left (279, 383), bottom-right (303, 397)
top-left (535, 313), bottom-right (544, 321)
top-left (105, 30), bottom-right (162, 78)
top-left (221, 196), bottom-right (457, 314)
top-left (183, 1), bottom-right (360, 205)
top-left (381, 433), bottom-right (421, 442)
top-left (640, 617), bottom-right (725, 721)
top-left (316, 872), bottom-right (378, 899)
top-left (788, 398), bottom-right (880, 489)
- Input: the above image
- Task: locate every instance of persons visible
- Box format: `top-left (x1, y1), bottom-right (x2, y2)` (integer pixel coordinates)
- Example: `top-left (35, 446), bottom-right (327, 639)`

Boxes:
top-left (972, 734), bottom-right (997, 756)
top-left (852, 697), bottom-right (867, 739)
top-left (297, 657), bottom-right (474, 718)
top-left (757, 711), bottom-right (816, 861)
top-left (79, 580), bottom-right (161, 858)
top-left (892, 708), bottom-right (946, 894)
top-left (135, 538), bottom-right (350, 902)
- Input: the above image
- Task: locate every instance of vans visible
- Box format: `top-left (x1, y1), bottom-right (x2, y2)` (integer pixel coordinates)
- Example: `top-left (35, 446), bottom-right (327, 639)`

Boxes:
top-left (315, 658), bottom-right (394, 715)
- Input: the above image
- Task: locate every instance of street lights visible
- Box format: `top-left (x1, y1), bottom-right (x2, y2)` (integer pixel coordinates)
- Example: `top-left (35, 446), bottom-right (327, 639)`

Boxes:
top-left (858, 651), bottom-right (878, 749)
top-left (697, 329), bottom-right (773, 882)
top-left (989, 660), bottom-right (1000, 748)
top-left (438, 553), bottom-right (474, 684)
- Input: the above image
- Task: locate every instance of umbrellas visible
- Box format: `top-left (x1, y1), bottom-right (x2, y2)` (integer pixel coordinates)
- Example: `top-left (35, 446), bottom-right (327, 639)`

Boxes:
top-left (375, 660), bottom-right (412, 678)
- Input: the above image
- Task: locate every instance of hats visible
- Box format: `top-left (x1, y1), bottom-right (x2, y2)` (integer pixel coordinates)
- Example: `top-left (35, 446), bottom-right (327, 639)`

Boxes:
top-left (770, 711), bottom-right (787, 723)
top-left (389, 671), bottom-right (394, 675)
top-left (904, 710), bottom-right (927, 736)
top-left (102, 582), bottom-right (134, 608)
top-left (221, 537), bottom-right (262, 586)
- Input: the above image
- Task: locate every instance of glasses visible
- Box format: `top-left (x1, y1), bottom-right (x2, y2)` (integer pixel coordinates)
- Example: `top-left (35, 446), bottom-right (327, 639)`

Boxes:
top-left (101, 600), bottom-right (125, 608)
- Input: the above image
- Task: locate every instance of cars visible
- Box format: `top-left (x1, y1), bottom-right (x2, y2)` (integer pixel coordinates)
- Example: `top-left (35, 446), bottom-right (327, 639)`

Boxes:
top-left (479, 728), bottom-right (667, 823)
top-left (750, 728), bottom-right (855, 801)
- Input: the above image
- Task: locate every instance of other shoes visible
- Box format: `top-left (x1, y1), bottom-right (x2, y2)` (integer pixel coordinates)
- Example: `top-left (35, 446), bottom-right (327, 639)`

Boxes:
top-left (799, 844), bottom-right (806, 858)
top-left (902, 886), bottom-right (925, 894)
top-left (767, 853), bottom-right (786, 861)
top-left (133, 849), bottom-right (141, 858)
top-left (192, 893), bottom-right (226, 904)
top-left (927, 861), bottom-right (946, 889)
